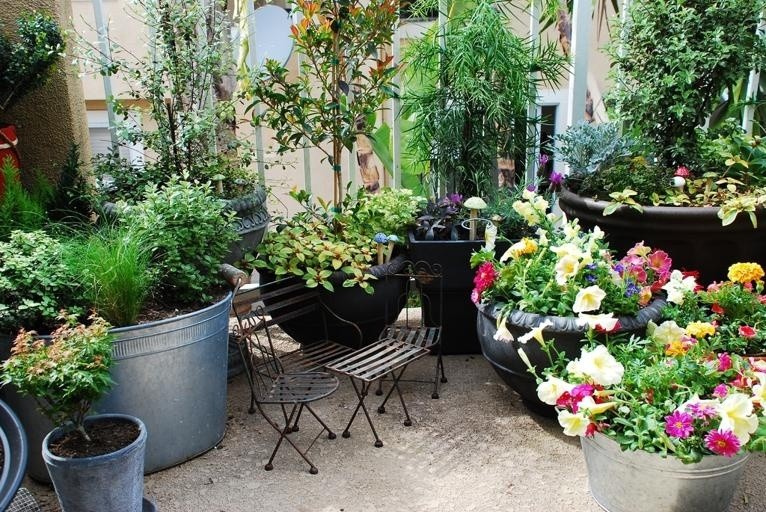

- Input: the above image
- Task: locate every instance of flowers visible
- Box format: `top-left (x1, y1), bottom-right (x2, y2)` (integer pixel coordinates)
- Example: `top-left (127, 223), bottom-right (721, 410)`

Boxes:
top-left (444, 151), bottom-right (766, 464)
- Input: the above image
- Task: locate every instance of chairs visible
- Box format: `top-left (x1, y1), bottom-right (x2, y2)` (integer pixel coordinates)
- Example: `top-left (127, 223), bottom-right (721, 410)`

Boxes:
top-left (232, 259), bottom-right (443, 475)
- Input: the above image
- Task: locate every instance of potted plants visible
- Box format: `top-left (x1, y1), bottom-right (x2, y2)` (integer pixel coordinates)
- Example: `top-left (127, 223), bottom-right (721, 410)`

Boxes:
top-left (3, 310), bottom-right (153, 511)
top-left (560, 1), bottom-right (765, 295)
top-left (59, 1), bottom-right (575, 365)
top-left (0, 177), bottom-right (243, 482)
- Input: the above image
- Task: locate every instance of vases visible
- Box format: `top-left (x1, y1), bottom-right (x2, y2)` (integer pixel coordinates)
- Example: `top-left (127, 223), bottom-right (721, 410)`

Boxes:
top-left (580, 422), bottom-right (753, 512)
